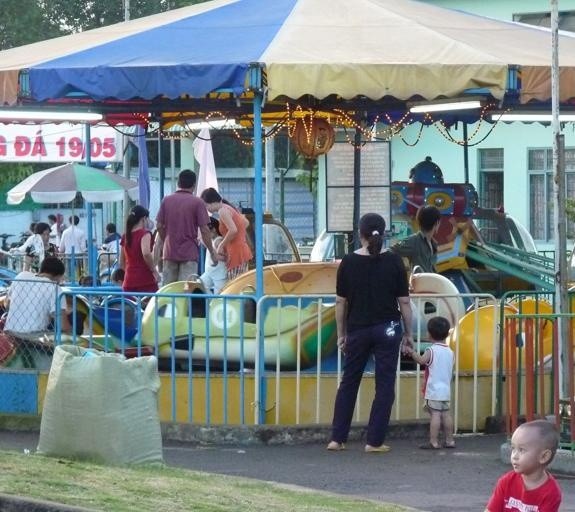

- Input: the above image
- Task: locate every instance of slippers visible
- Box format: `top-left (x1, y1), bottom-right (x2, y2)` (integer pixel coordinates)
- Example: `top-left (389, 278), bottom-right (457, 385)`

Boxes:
top-left (365, 444), bottom-right (391, 453)
top-left (443, 441), bottom-right (456, 448)
top-left (419, 441), bottom-right (440, 450)
top-left (328, 441), bottom-right (345, 451)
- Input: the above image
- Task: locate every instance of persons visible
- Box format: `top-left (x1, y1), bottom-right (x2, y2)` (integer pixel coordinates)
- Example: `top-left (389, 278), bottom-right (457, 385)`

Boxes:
top-left (4, 256), bottom-right (71, 334)
top-left (384, 205), bottom-right (441, 277)
top-left (402, 316), bottom-right (459, 450)
top-left (79, 268), bottom-right (125, 286)
top-left (483, 417), bottom-right (562, 512)
top-left (2, 167), bottom-right (253, 257)
top-left (326, 213), bottom-right (411, 454)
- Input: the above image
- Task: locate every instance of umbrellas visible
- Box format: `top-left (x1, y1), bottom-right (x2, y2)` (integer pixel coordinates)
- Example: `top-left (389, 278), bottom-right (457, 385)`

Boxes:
top-left (5, 163), bottom-right (140, 253)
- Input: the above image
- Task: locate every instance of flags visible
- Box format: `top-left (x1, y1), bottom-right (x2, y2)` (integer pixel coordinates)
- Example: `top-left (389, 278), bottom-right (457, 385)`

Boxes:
top-left (193, 126), bottom-right (219, 218)
top-left (132, 126), bottom-right (151, 214)
